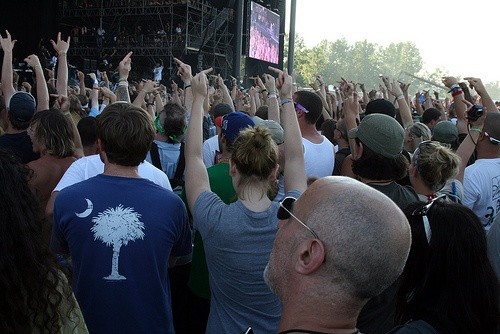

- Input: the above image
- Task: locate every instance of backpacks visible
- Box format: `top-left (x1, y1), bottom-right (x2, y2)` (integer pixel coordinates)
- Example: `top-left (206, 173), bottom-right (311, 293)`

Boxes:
top-left (150, 142), bottom-right (194, 229)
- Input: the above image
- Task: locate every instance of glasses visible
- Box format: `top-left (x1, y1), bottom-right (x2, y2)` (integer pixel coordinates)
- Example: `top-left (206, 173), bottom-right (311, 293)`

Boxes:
top-left (418, 139), bottom-right (432, 153)
top-left (413, 190), bottom-right (462, 245)
top-left (407, 123), bottom-right (412, 131)
top-left (484, 132), bottom-right (500, 145)
top-left (277, 196), bottom-right (321, 239)
top-left (334, 124), bottom-right (343, 136)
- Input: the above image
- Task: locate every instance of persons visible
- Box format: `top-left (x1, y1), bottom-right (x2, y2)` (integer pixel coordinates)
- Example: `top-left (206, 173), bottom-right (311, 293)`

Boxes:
top-left (0, 30), bottom-right (500, 334)
top-left (243, 176), bottom-right (412, 334)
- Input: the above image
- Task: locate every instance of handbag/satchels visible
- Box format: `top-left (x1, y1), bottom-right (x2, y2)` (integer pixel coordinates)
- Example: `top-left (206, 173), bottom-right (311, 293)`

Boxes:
top-left (18, 257), bottom-right (89, 334)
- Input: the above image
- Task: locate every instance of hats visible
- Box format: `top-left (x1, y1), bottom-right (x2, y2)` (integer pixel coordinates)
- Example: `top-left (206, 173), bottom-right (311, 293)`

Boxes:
top-left (213, 102), bottom-right (233, 117)
top-left (220, 112), bottom-right (255, 138)
top-left (9, 91), bottom-right (37, 122)
top-left (359, 98), bottom-right (396, 117)
top-left (431, 121), bottom-right (459, 141)
top-left (346, 114), bottom-right (405, 156)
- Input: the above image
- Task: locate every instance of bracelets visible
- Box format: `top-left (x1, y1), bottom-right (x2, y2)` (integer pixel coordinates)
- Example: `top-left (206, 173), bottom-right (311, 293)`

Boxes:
top-left (118, 78), bottom-right (129, 87)
top-left (34, 64), bottom-right (42, 68)
top-left (154, 92), bottom-right (159, 97)
top-left (448, 84), bottom-right (463, 96)
top-left (396, 95), bottom-right (404, 100)
top-left (261, 89), bottom-right (267, 92)
top-left (147, 103), bottom-right (154, 106)
top-left (185, 84), bottom-right (191, 90)
top-left (470, 127), bottom-right (482, 133)
top-left (362, 90), bottom-right (366, 92)
top-left (268, 95), bottom-right (278, 98)
top-left (140, 88), bottom-right (147, 93)
top-left (233, 84), bottom-right (236, 86)
top-left (93, 80), bottom-right (98, 84)
top-left (281, 97), bottom-right (292, 105)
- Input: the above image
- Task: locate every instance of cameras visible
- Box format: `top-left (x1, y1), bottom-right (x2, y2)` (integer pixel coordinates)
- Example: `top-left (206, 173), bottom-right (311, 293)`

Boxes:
top-left (466, 105), bottom-right (484, 120)
